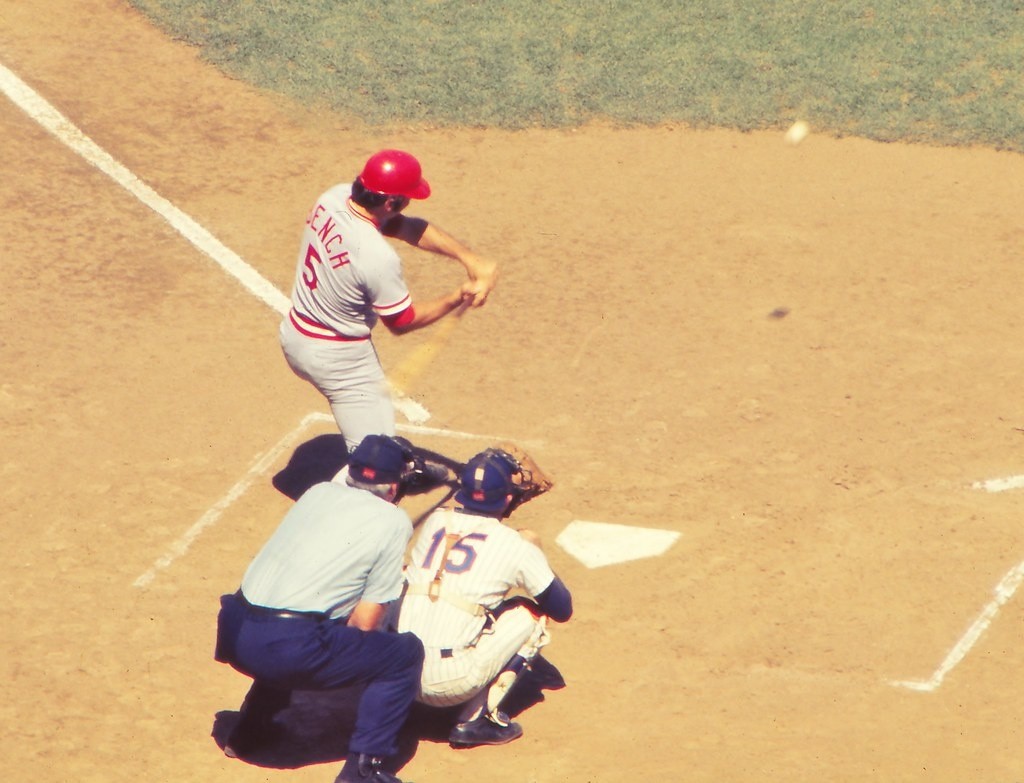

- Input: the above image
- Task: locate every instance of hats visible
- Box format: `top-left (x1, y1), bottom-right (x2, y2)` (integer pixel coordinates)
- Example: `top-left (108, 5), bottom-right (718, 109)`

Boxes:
top-left (348, 435), bottom-right (416, 483)
top-left (455, 450), bottom-right (515, 512)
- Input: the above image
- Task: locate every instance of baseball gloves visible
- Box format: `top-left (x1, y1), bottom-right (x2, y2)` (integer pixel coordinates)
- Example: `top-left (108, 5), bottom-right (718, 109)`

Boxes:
top-left (490, 440), bottom-right (551, 498)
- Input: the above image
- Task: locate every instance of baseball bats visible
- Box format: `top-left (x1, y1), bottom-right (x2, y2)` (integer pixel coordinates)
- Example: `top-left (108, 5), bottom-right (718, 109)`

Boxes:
top-left (385, 294), bottom-right (475, 399)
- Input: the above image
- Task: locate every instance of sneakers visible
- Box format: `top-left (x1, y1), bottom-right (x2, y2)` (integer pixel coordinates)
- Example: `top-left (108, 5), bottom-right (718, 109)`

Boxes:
top-left (226, 715), bottom-right (282, 758)
top-left (334, 753), bottom-right (402, 783)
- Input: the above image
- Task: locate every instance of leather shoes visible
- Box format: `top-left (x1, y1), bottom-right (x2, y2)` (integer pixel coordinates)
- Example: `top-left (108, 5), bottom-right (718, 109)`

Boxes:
top-left (448, 715), bottom-right (523, 745)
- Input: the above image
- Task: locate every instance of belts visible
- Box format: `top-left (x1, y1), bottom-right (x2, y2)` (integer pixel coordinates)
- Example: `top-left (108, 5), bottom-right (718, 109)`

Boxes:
top-left (236, 590), bottom-right (327, 623)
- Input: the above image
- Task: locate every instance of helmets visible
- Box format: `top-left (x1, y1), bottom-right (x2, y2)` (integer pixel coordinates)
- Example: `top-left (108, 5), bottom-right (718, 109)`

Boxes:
top-left (358, 149), bottom-right (431, 199)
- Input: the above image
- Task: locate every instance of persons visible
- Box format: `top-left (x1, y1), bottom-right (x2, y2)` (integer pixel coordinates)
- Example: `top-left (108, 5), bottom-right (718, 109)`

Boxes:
top-left (277, 147), bottom-right (500, 488)
top-left (381, 446), bottom-right (573, 745)
top-left (215, 432), bottom-right (426, 783)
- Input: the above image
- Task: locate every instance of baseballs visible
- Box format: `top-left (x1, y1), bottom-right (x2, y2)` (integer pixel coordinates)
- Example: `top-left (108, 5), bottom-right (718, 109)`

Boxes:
top-left (784, 119), bottom-right (812, 145)
